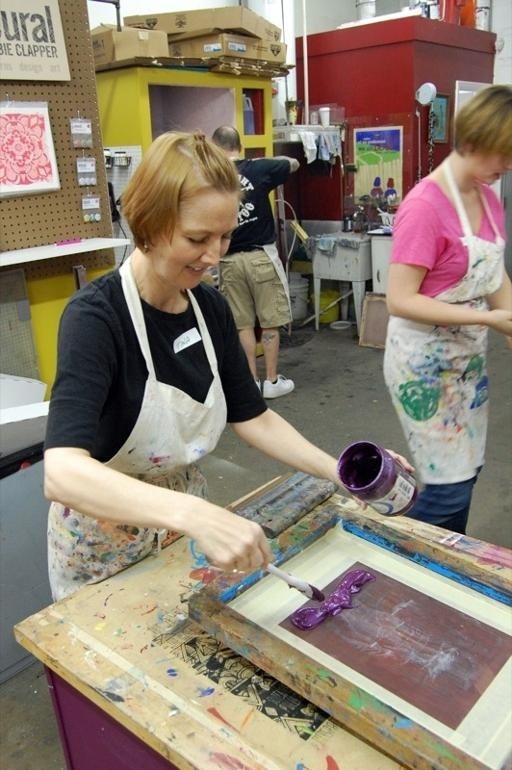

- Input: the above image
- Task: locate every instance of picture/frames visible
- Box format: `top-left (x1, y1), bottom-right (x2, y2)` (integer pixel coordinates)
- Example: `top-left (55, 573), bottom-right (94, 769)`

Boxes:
top-left (429, 92), bottom-right (451, 144)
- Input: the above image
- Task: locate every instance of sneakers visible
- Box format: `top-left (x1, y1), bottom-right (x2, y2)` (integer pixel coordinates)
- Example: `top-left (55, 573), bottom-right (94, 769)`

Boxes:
top-left (263, 375), bottom-right (295, 399)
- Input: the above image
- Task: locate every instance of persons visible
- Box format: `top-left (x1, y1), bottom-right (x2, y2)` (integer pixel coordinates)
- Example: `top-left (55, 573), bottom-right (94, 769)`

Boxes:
top-left (380, 84), bottom-right (512, 535)
top-left (38, 126), bottom-right (412, 606)
top-left (200, 123), bottom-right (300, 400)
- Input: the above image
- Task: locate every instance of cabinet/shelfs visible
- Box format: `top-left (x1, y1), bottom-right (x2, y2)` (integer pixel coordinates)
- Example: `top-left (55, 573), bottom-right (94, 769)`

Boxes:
top-left (95, 67), bottom-right (280, 357)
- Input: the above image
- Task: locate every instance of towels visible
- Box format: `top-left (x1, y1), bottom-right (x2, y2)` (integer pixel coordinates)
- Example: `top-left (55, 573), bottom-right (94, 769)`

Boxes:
top-left (298, 131), bottom-right (339, 164)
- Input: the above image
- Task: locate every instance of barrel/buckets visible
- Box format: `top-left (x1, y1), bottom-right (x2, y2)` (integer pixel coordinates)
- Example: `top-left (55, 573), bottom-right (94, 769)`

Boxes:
top-left (243, 94), bottom-right (255, 135)
top-left (287, 278), bottom-right (309, 320)
top-left (356, 0), bottom-right (376, 20)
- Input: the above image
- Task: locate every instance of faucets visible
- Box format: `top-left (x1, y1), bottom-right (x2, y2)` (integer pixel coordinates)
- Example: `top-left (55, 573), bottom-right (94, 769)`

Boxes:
top-left (353, 211), bottom-right (371, 231)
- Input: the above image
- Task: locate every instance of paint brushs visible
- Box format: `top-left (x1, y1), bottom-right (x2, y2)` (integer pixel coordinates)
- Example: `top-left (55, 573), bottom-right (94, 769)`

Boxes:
top-left (266, 564), bottom-right (324, 601)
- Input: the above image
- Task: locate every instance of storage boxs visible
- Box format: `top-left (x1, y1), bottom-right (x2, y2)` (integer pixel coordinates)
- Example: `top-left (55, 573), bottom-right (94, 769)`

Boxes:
top-left (90, 23), bottom-right (170, 70)
top-left (168, 33), bottom-right (286, 65)
top-left (123, 6), bottom-right (283, 47)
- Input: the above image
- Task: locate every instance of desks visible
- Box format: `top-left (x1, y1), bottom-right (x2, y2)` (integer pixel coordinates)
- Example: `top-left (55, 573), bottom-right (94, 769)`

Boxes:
top-left (13, 471), bottom-right (510, 770)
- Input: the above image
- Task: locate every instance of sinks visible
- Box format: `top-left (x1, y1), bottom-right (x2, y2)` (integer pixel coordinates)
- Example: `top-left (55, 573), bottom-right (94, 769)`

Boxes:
top-left (310, 232), bottom-right (372, 282)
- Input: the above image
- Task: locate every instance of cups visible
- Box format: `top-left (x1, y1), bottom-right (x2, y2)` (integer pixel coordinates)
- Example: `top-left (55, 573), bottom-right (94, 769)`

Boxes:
top-left (284, 100), bottom-right (298, 126)
top-left (336, 440), bottom-right (418, 518)
top-left (319, 107), bottom-right (330, 126)
